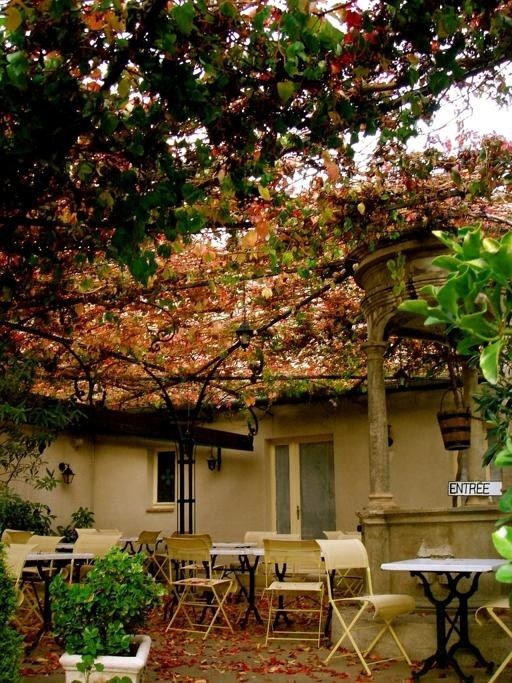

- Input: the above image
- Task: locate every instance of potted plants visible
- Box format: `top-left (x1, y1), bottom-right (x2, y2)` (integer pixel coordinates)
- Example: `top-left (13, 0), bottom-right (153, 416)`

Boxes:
top-left (49, 548), bottom-right (167, 681)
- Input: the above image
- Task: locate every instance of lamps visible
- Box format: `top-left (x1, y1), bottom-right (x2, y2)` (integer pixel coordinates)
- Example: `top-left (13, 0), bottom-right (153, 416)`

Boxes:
top-left (59, 462), bottom-right (75, 484)
top-left (207, 446), bottom-right (221, 471)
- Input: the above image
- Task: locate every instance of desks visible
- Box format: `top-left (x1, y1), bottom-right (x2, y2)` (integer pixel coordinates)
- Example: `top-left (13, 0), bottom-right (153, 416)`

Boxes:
top-left (197, 546), bottom-right (265, 627)
top-left (210, 540), bottom-right (256, 606)
top-left (380, 557), bottom-right (508, 683)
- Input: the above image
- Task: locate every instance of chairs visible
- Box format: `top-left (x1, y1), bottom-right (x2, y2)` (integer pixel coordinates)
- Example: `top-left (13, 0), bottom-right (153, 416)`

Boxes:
top-left (164, 537), bottom-right (233, 641)
top-left (323, 528), bottom-right (363, 539)
top-left (473, 597), bottom-right (512, 681)
top-left (314, 539), bottom-right (416, 678)
top-left (242, 531), bottom-right (277, 599)
top-left (2, 527), bottom-right (164, 657)
top-left (262, 538), bottom-right (327, 647)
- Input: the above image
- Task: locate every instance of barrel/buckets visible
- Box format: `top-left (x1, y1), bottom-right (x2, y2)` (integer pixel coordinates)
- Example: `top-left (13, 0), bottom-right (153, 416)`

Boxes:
top-left (436, 388), bottom-right (471, 451)
